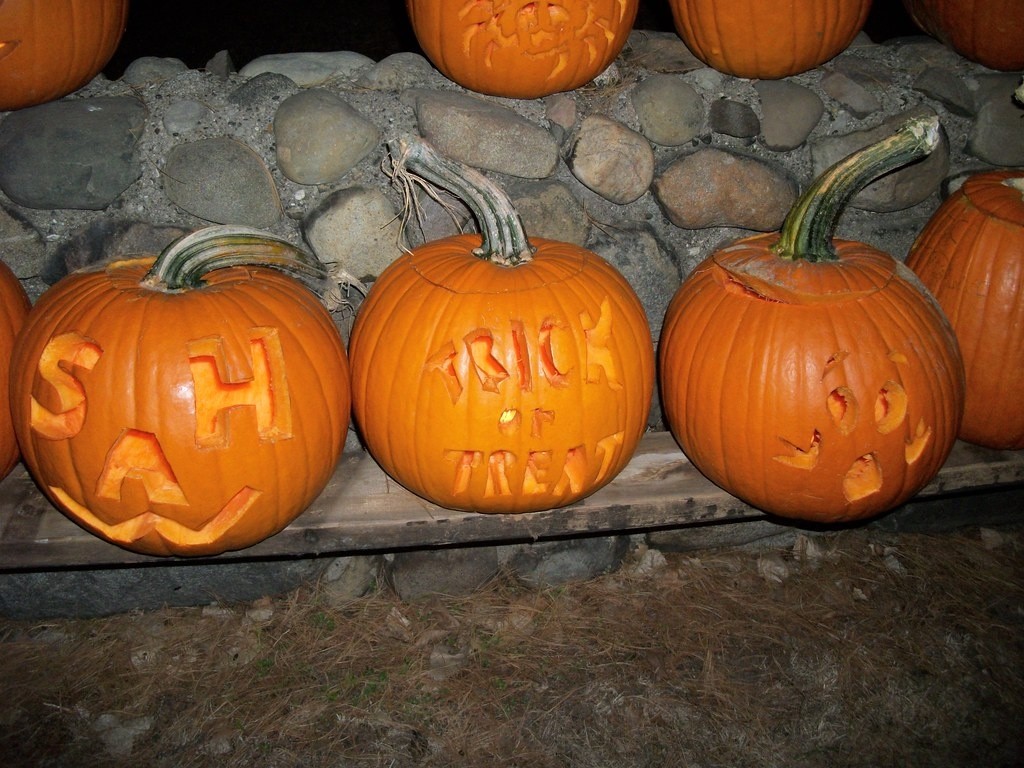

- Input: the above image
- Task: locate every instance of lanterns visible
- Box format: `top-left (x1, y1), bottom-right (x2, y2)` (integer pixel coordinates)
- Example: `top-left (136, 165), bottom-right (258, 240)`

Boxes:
top-left (407, 0), bottom-right (645, 99)
top-left (10, 219), bottom-right (352, 566)
top-left (657, 117), bottom-right (966, 524)
top-left (344, 136), bottom-right (656, 516)
top-left (0, 1), bottom-right (125, 109)
